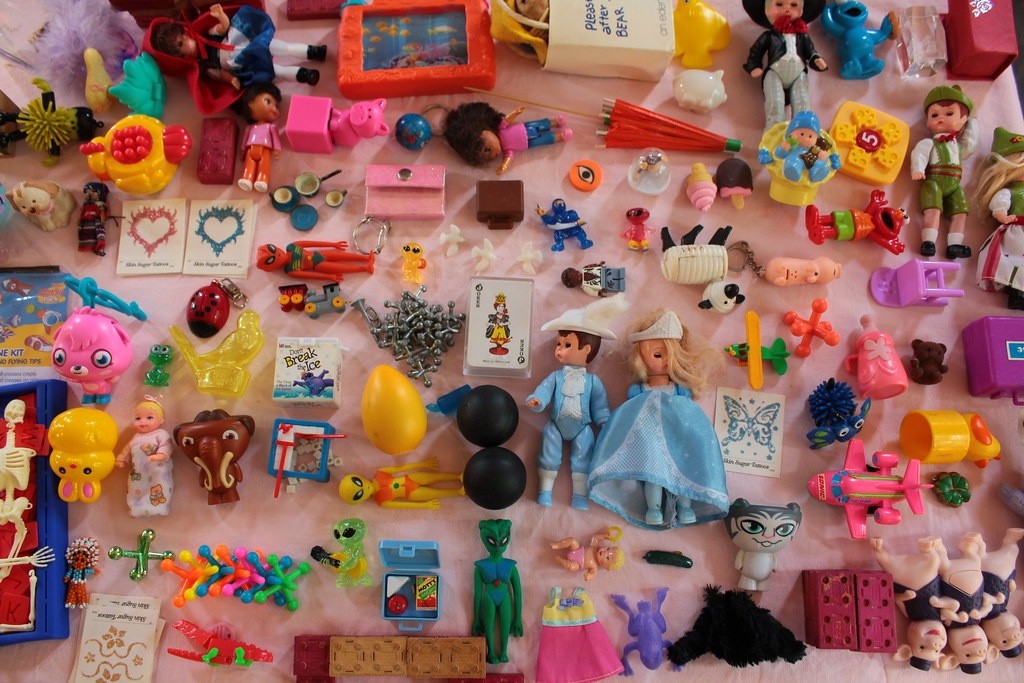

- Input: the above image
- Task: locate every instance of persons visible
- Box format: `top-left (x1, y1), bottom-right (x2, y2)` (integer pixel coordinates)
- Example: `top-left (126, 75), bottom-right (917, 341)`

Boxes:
top-left (441, 99), bottom-right (571, 173)
top-left (114, 401), bottom-right (174, 519)
top-left (738, 0), bottom-right (829, 136)
top-left (584, 306), bottom-right (729, 532)
top-left (909, 83), bottom-right (972, 259)
top-left (972, 126), bottom-right (1024, 310)
top-left (774, 110), bottom-right (831, 183)
top-left (228, 76), bottom-right (285, 191)
top-left (61, 538), bottom-right (100, 610)
top-left (141, 2), bottom-right (329, 111)
top-left (523, 307), bottom-right (616, 510)
top-left (549, 525), bottom-right (624, 581)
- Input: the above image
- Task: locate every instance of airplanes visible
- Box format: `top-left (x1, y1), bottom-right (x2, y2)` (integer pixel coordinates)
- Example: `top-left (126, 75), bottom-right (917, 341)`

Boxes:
top-left (807, 437), bottom-right (933, 540)
